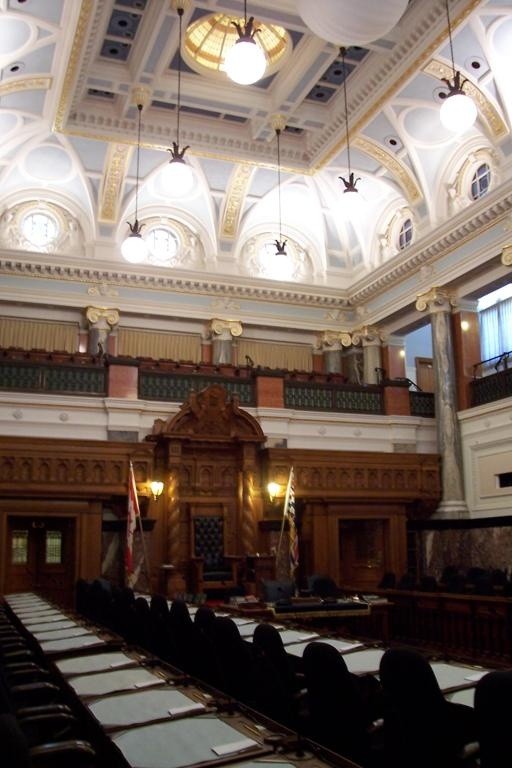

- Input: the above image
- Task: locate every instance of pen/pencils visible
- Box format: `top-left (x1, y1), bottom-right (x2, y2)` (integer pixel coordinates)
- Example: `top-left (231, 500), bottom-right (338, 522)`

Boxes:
top-left (193, 692), bottom-right (206, 702)
top-left (132, 651), bottom-right (141, 659)
top-left (243, 724), bottom-right (262, 737)
top-left (255, 760), bottom-right (288, 764)
top-left (159, 671), bottom-right (166, 678)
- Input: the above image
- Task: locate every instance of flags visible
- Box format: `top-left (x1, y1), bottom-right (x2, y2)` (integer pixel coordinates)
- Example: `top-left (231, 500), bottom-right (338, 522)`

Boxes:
top-left (285, 471), bottom-right (299, 577)
top-left (123, 466), bottom-right (145, 590)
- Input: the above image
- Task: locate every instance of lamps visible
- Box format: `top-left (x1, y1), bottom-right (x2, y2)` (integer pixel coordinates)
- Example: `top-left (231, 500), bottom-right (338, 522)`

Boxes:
top-left (270, 116), bottom-right (298, 276)
top-left (437, 4), bottom-right (477, 135)
top-left (224, 2), bottom-right (267, 85)
top-left (150, 480), bottom-right (164, 502)
top-left (267, 482), bottom-right (281, 504)
top-left (160, 8), bottom-right (196, 197)
top-left (120, 101), bottom-right (151, 264)
top-left (337, 46), bottom-right (362, 212)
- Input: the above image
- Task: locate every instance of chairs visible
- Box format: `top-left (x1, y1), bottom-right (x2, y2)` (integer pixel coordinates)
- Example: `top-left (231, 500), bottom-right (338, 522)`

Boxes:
top-left (0, 566), bottom-right (512, 768)
